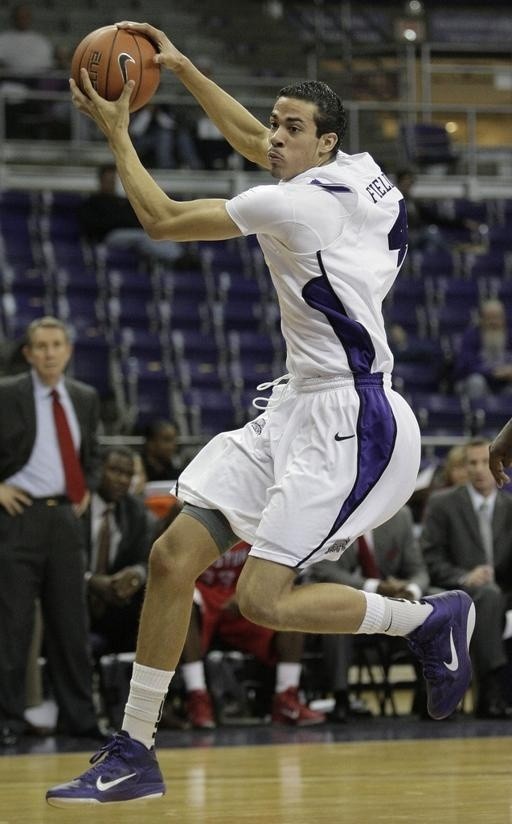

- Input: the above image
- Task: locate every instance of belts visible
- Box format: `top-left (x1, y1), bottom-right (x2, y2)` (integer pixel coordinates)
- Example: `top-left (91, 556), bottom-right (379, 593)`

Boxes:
top-left (35, 496), bottom-right (68, 507)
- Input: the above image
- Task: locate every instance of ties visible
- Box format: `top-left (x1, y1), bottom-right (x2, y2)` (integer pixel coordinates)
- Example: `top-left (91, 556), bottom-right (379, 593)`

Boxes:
top-left (357, 535), bottom-right (381, 579)
top-left (89, 509), bottom-right (110, 623)
top-left (477, 503), bottom-right (494, 572)
top-left (49, 389), bottom-right (84, 502)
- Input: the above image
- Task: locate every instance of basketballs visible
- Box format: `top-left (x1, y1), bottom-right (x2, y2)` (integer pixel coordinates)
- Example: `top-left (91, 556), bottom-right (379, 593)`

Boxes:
top-left (70, 24), bottom-right (160, 113)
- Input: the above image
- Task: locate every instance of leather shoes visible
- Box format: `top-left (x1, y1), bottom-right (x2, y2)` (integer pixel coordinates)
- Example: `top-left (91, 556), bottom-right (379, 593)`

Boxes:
top-left (0, 720), bottom-right (18, 747)
top-left (90, 717), bottom-right (120, 742)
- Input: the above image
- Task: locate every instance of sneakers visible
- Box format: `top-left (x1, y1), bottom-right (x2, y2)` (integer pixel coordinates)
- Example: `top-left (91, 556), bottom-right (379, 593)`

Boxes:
top-left (184, 689), bottom-right (217, 729)
top-left (46, 730), bottom-right (165, 810)
top-left (158, 708), bottom-right (191, 730)
top-left (270, 686), bottom-right (327, 728)
top-left (476, 703), bottom-right (512, 719)
top-left (406, 589), bottom-right (476, 721)
top-left (332, 700), bottom-right (351, 724)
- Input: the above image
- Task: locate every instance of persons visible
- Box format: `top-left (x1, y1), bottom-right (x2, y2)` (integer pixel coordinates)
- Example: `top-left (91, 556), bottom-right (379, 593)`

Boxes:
top-left (73, 159), bottom-right (206, 272)
top-left (2, 311), bottom-right (116, 753)
top-left (1, 2), bottom-right (71, 139)
top-left (452, 295), bottom-right (510, 427)
top-left (27, 415), bottom-right (512, 739)
top-left (196, 111), bottom-right (260, 172)
top-left (128, 81), bottom-right (203, 171)
top-left (397, 168), bottom-right (491, 256)
top-left (44, 17), bottom-right (481, 813)
